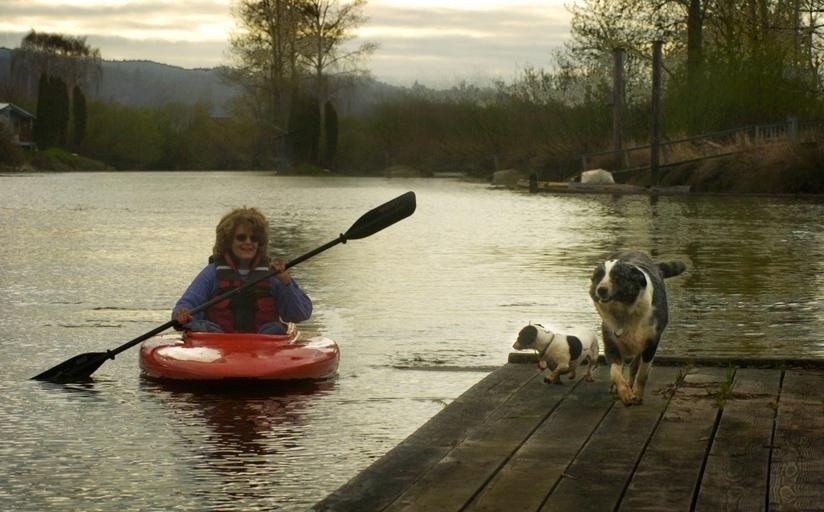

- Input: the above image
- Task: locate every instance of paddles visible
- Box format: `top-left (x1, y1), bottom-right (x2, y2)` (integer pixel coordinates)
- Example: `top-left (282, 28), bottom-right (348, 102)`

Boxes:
top-left (29, 191), bottom-right (416, 383)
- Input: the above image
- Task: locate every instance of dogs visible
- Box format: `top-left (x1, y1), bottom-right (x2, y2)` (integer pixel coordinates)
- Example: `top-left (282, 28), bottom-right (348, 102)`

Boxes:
top-left (590, 250), bottom-right (686, 406)
top-left (513, 324), bottom-right (599, 385)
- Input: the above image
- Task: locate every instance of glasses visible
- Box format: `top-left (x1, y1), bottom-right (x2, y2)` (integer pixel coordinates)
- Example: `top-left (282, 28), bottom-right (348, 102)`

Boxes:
top-left (232, 233), bottom-right (261, 243)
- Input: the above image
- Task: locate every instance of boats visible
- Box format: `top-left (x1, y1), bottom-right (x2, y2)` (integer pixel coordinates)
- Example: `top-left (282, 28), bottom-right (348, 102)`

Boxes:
top-left (136, 333), bottom-right (344, 385)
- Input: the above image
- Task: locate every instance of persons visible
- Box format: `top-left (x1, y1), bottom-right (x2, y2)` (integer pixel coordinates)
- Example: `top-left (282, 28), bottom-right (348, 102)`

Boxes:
top-left (172, 204), bottom-right (313, 334)
